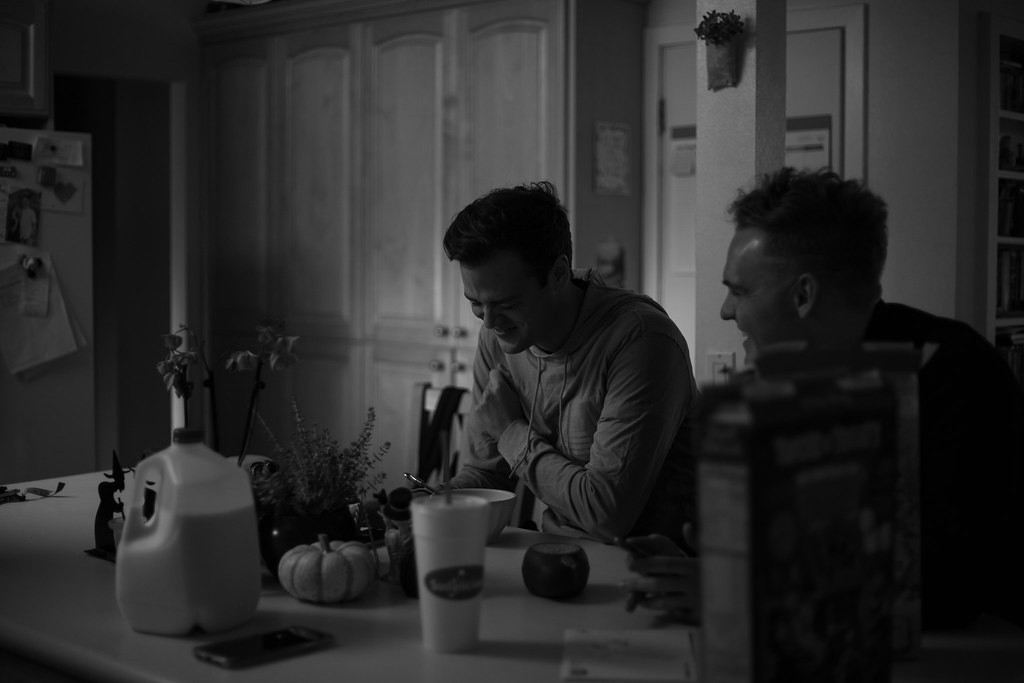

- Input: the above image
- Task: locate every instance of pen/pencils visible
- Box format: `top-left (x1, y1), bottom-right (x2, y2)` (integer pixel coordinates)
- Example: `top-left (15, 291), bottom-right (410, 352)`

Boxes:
top-left (613, 537), bottom-right (649, 557)
top-left (402, 472), bottom-right (440, 496)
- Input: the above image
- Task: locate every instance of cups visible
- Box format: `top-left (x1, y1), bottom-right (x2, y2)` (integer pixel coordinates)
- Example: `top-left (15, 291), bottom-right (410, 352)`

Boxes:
top-left (410, 494), bottom-right (492, 654)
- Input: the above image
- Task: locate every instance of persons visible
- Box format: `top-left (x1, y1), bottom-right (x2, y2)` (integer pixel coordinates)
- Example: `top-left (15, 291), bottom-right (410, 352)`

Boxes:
top-left (620, 168), bottom-right (1024, 634)
top-left (361, 182), bottom-right (702, 547)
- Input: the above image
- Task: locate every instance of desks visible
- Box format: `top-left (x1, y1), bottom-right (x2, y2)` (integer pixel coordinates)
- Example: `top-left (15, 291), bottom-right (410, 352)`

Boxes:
top-left (0, 466), bottom-right (1024, 683)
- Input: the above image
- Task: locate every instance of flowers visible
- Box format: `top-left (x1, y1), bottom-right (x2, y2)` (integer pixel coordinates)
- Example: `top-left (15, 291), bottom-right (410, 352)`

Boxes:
top-left (245, 391), bottom-right (392, 517)
top-left (695, 9), bottom-right (744, 47)
top-left (157, 323), bottom-right (304, 467)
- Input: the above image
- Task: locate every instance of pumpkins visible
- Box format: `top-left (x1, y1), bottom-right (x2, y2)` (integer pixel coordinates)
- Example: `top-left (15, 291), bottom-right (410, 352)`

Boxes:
top-left (278, 533), bottom-right (376, 604)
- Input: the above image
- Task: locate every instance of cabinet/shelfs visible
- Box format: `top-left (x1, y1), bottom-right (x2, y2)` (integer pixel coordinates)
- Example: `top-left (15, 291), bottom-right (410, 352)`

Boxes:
top-left (193, 0), bottom-right (648, 503)
top-left (977, 14), bottom-right (1024, 386)
top-left (0, 0), bottom-right (52, 118)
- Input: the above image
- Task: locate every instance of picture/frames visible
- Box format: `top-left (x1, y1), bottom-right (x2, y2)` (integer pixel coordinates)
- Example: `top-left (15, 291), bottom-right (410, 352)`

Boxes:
top-left (593, 120), bottom-right (633, 199)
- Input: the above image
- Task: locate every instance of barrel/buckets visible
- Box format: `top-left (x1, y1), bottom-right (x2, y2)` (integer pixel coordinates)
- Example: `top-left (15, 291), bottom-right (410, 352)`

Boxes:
top-left (113, 426), bottom-right (262, 636)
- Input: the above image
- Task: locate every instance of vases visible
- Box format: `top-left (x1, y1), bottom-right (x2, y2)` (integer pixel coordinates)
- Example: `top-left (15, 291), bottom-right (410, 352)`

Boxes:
top-left (259, 507), bottom-right (356, 576)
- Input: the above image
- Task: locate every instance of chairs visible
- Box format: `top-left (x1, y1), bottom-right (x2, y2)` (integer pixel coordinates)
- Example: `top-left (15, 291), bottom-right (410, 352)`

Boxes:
top-left (403, 381), bottom-right (536, 528)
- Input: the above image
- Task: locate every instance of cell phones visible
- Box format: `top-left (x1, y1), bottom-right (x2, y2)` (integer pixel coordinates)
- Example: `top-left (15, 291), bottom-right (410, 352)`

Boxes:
top-left (193, 624), bottom-right (338, 670)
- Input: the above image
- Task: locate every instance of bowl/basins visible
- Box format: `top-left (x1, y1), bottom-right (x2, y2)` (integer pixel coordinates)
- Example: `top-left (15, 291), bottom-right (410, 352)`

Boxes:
top-left (433, 488), bottom-right (517, 545)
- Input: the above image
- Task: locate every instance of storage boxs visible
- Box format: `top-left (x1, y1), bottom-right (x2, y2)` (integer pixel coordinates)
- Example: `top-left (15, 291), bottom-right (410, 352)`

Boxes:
top-left (697, 364), bottom-right (900, 683)
top-left (759, 341), bottom-right (939, 658)
